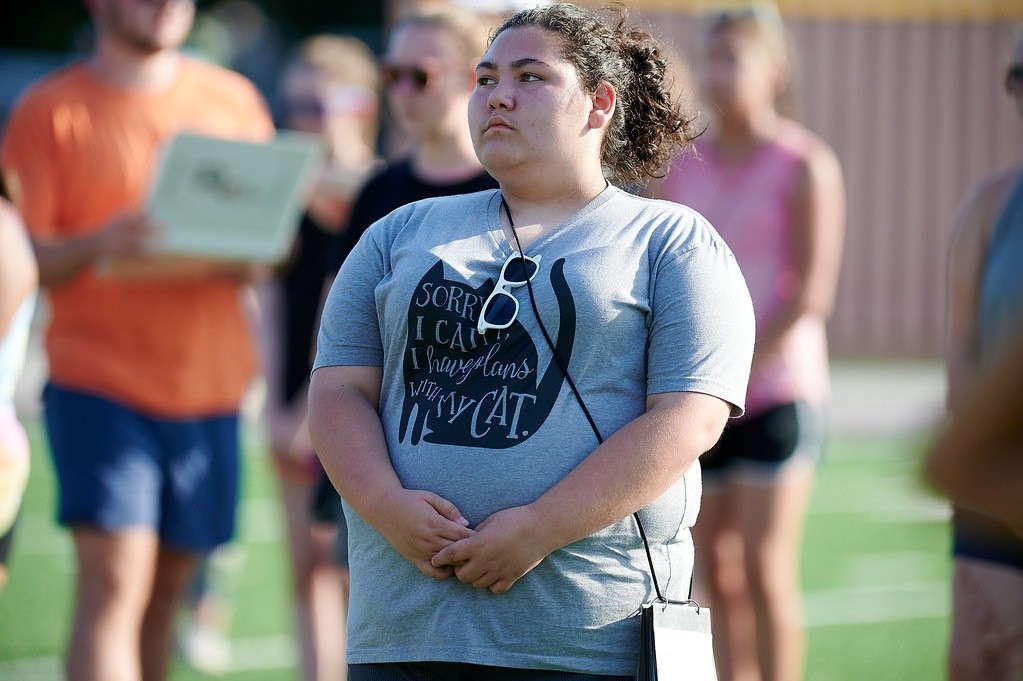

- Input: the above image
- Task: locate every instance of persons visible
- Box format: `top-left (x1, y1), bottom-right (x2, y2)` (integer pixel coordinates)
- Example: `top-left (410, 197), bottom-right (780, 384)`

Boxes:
top-left (0, 0), bottom-right (499, 681)
top-left (647, 1), bottom-right (847, 681)
top-left (924, 57), bottom-right (1023, 681)
top-left (306, 0), bottom-right (757, 681)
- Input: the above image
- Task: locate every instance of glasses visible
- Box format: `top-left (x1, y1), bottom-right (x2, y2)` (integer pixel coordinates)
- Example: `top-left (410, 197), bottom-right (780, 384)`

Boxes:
top-left (381, 62), bottom-right (471, 94)
top-left (478, 251), bottom-right (542, 334)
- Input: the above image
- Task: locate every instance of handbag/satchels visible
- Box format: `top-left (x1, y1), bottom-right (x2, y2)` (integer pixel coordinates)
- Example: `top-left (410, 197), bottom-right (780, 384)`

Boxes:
top-left (638, 597), bottom-right (719, 681)
